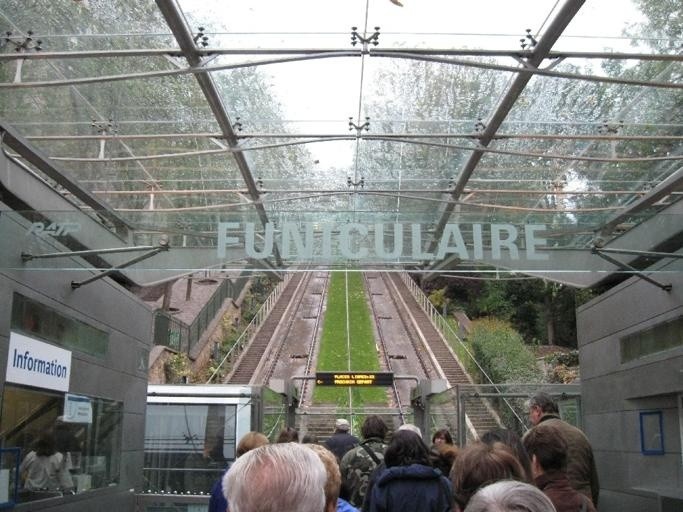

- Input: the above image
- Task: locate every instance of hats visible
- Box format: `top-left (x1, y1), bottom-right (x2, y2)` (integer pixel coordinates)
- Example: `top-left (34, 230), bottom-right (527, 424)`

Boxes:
top-left (334, 418), bottom-right (350, 432)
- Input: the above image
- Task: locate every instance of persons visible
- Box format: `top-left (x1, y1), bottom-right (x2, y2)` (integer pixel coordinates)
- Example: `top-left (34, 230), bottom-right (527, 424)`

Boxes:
top-left (15, 412), bottom-right (106, 497)
